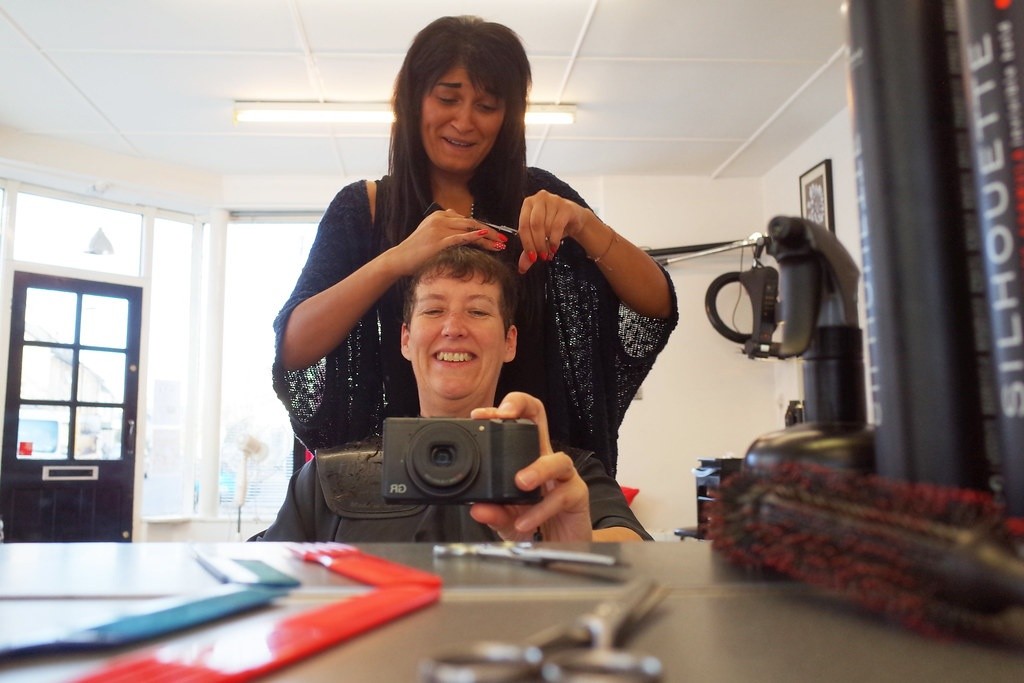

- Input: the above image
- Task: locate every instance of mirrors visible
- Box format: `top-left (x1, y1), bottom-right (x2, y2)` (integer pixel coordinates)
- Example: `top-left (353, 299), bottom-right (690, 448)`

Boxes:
top-left (2, 0), bottom-right (1024, 587)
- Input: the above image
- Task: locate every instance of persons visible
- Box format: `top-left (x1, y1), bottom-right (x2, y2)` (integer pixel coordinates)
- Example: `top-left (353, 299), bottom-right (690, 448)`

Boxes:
top-left (273, 18), bottom-right (681, 487)
top-left (254, 243), bottom-right (654, 540)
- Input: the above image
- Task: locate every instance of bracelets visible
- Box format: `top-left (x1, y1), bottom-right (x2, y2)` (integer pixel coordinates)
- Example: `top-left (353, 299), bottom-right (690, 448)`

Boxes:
top-left (585, 225), bottom-right (620, 271)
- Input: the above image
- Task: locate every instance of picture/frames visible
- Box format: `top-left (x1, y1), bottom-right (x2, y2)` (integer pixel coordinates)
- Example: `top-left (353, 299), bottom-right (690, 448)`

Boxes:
top-left (799, 160), bottom-right (834, 236)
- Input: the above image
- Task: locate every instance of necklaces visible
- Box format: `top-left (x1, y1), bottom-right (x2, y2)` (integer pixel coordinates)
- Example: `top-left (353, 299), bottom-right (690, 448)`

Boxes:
top-left (470, 204), bottom-right (475, 220)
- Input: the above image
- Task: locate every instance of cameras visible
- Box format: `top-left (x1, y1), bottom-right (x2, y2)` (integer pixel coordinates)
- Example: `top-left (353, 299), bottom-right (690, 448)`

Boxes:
top-left (381, 417), bottom-right (542, 506)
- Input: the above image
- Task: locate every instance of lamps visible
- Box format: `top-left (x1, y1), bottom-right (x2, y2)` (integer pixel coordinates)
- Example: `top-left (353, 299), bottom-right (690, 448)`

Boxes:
top-left (233, 101), bottom-right (577, 126)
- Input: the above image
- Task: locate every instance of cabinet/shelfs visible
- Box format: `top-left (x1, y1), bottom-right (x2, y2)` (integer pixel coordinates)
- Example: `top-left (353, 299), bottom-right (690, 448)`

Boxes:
top-left (694, 457), bottom-right (743, 538)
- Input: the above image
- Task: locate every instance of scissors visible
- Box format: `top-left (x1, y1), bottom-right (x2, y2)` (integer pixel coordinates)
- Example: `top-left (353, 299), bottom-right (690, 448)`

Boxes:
top-left (475, 218), bottom-right (564, 245)
top-left (432, 538), bottom-right (619, 567)
top-left (418, 575), bottom-right (675, 683)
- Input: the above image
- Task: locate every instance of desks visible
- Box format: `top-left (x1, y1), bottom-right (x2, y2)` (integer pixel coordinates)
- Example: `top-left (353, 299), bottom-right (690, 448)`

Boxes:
top-left (0, 588), bottom-right (1024, 683)
top-left (0, 532), bottom-right (761, 589)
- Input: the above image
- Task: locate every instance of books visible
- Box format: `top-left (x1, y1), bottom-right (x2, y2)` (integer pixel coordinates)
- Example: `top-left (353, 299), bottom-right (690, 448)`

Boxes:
top-left (848, 0), bottom-right (1024, 523)
